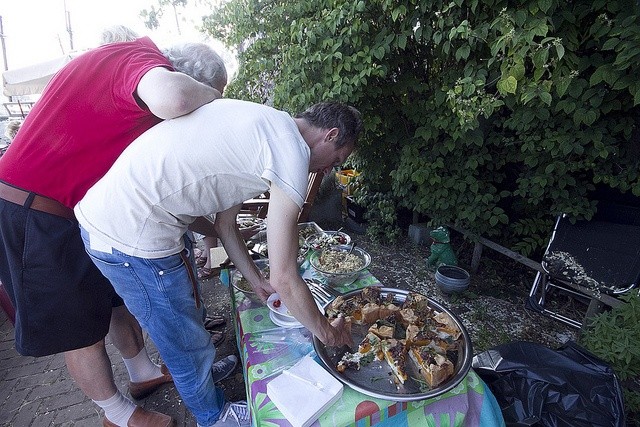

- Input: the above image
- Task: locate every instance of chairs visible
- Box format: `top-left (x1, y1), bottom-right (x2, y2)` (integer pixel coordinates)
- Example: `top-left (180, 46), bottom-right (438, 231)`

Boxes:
top-left (531, 212), bottom-right (638, 326)
top-left (234, 170), bottom-right (324, 232)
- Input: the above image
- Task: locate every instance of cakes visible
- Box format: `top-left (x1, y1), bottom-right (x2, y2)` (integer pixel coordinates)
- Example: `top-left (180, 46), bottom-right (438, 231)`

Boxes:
top-left (326, 286), bottom-right (462, 389)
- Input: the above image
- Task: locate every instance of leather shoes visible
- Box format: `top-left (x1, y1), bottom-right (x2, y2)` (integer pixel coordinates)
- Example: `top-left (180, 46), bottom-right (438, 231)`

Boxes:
top-left (128, 364), bottom-right (174, 401)
top-left (102, 406), bottom-right (174, 427)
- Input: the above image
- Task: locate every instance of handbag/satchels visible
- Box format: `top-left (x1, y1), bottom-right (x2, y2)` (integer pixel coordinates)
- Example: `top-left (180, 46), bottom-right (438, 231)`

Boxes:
top-left (471, 340), bottom-right (625, 427)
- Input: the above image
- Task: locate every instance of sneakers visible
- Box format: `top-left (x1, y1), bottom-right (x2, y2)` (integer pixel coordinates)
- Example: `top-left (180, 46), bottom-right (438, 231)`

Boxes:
top-left (197, 401), bottom-right (249, 427)
top-left (212, 355), bottom-right (238, 383)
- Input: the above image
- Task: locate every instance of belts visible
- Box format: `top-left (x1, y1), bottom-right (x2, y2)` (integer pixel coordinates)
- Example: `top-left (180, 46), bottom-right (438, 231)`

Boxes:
top-left (180, 251), bottom-right (201, 310)
top-left (0, 180), bottom-right (77, 224)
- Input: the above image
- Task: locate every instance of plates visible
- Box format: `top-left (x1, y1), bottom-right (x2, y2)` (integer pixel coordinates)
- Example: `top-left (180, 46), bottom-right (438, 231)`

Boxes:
top-left (269, 298), bottom-right (325, 328)
top-left (313, 286), bottom-right (473, 403)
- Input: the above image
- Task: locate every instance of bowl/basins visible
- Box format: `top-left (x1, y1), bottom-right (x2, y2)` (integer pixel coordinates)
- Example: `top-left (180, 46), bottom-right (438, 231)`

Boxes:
top-left (237, 218), bottom-right (264, 239)
top-left (305, 231), bottom-right (351, 251)
top-left (267, 293), bottom-right (295, 322)
top-left (310, 245), bottom-right (371, 286)
top-left (231, 259), bottom-right (276, 306)
top-left (258, 242), bottom-right (310, 270)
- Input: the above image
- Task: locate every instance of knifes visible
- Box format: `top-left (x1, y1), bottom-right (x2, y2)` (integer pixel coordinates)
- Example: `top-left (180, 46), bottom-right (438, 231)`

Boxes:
top-left (306, 282), bottom-right (330, 303)
top-left (305, 278), bottom-right (332, 298)
top-left (312, 278), bottom-right (342, 297)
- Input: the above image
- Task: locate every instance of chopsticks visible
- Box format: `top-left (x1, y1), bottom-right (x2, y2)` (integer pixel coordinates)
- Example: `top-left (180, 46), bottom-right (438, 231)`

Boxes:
top-left (252, 206), bottom-right (263, 222)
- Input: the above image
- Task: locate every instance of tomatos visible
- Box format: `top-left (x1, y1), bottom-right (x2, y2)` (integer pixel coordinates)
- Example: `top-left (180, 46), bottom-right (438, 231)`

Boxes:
top-left (272, 300), bottom-right (281, 307)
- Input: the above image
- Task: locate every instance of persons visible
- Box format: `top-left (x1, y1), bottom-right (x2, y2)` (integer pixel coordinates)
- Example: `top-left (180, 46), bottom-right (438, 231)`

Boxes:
top-left (202, 312), bottom-right (227, 348)
top-left (103, 26), bottom-right (140, 43)
top-left (0, 36), bottom-right (227, 426)
top-left (194, 213), bottom-right (221, 279)
top-left (5, 120), bottom-right (22, 140)
top-left (73, 97), bottom-right (363, 426)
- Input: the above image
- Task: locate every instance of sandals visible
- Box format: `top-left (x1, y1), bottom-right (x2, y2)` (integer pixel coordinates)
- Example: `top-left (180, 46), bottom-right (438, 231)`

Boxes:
top-left (196, 267), bottom-right (221, 280)
top-left (195, 255), bottom-right (207, 268)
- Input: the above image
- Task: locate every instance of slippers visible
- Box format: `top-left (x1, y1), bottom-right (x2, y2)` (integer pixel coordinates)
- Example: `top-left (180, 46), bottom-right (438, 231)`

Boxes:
top-left (209, 330), bottom-right (225, 347)
top-left (205, 315), bottom-right (225, 330)
